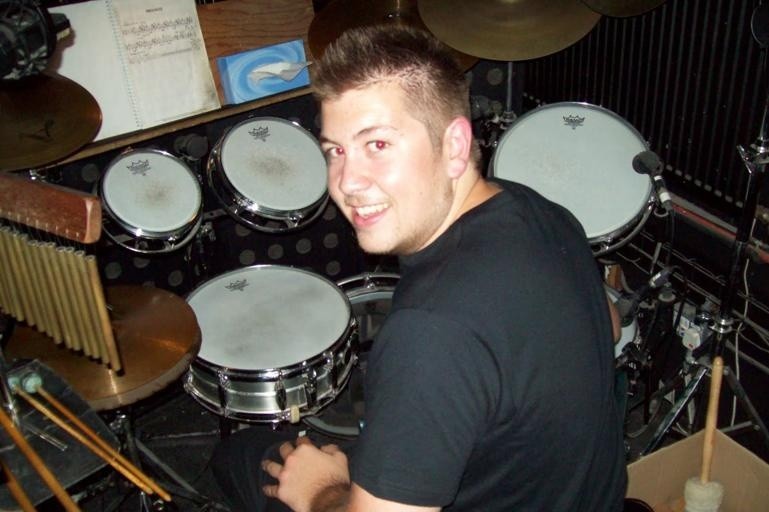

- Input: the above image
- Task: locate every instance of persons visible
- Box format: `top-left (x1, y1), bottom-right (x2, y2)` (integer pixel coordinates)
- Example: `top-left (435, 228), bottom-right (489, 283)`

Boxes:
top-left (208, 24), bottom-right (628, 512)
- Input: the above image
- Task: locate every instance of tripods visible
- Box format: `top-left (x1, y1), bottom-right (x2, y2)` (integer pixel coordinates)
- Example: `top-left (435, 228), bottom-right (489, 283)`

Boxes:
top-left (626, 137), bottom-right (769, 458)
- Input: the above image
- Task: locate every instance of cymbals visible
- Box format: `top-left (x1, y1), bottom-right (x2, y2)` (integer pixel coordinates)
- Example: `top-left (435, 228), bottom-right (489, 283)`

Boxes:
top-left (0, 70), bottom-right (101, 171)
top-left (418, 1), bottom-right (601, 62)
top-left (585, 0), bottom-right (665, 17)
top-left (5, 284), bottom-right (202, 413)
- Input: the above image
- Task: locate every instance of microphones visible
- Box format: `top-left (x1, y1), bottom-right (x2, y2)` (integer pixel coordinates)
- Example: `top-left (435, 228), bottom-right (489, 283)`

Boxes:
top-left (647, 267), bottom-right (674, 288)
top-left (174, 133), bottom-right (210, 159)
top-left (0, 0), bottom-right (70, 81)
top-left (632, 148), bottom-right (674, 213)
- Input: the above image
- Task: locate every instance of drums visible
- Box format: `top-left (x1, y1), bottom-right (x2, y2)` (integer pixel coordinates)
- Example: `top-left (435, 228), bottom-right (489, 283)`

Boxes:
top-left (185, 264), bottom-right (355, 423)
top-left (94, 147), bottom-right (204, 253)
top-left (206, 117), bottom-right (329, 232)
top-left (299, 274), bottom-right (404, 442)
top-left (490, 101), bottom-right (656, 257)
